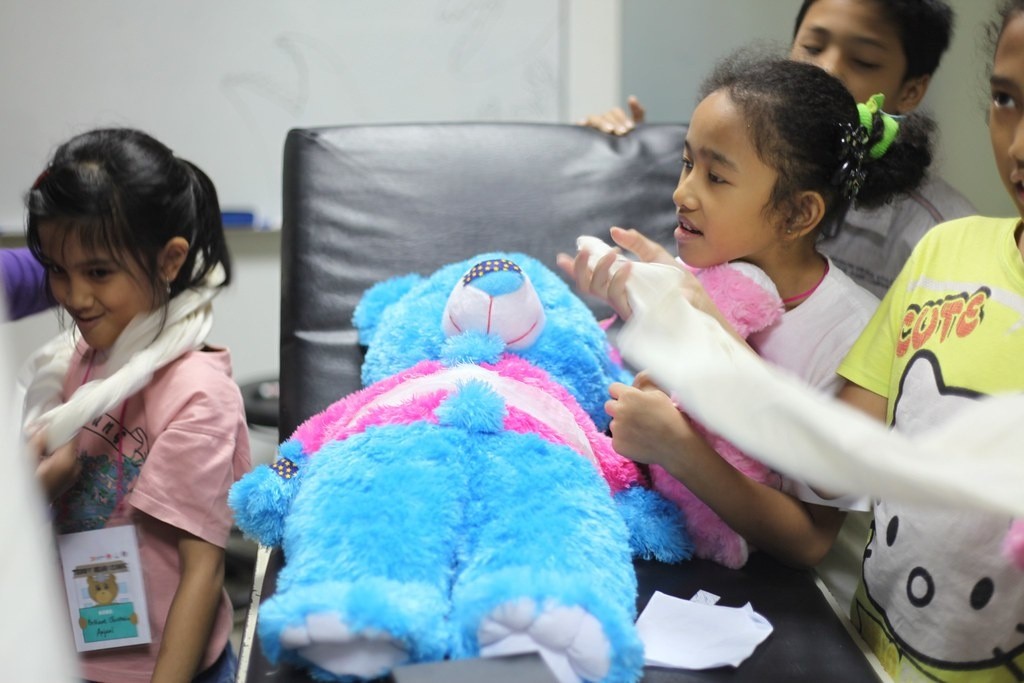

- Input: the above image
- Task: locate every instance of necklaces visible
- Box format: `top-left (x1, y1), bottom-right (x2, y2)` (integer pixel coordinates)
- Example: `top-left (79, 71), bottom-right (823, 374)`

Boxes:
top-left (782, 265), bottom-right (829, 303)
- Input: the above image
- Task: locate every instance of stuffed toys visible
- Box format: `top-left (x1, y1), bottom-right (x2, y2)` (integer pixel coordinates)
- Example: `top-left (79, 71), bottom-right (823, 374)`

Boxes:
top-left (599, 255), bottom-right (784, 570)
top-left (224, 248), bottom-right (691, 683)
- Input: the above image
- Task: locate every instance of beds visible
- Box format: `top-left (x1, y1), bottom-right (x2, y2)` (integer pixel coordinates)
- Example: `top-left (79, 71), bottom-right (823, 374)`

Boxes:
top-left (232, 123), bottom-right (894, 682)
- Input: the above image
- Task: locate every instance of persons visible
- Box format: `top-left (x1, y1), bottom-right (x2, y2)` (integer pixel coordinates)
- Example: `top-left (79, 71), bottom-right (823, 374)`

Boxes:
top-left (0, 128), bottom-right (253, 683)
top-left (552, 0), bottom-right (1024, 683)
